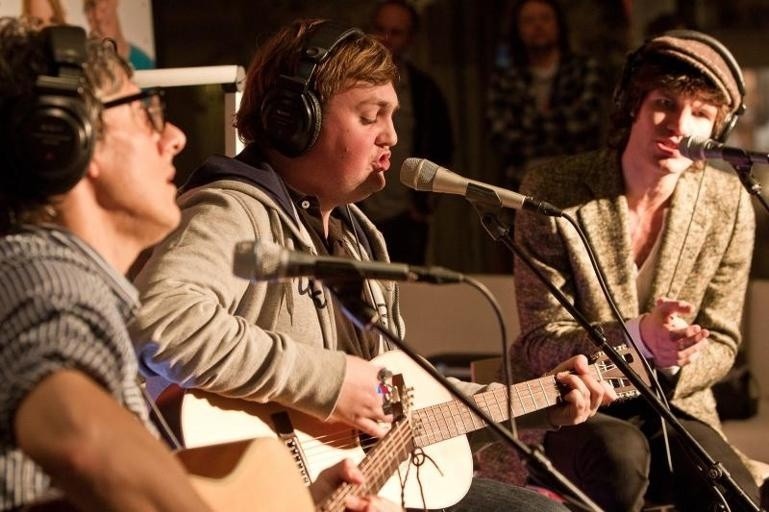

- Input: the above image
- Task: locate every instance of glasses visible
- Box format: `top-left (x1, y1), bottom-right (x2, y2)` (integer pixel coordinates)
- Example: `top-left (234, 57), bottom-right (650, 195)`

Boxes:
top-left (101, 86), bottom-right (169, 131)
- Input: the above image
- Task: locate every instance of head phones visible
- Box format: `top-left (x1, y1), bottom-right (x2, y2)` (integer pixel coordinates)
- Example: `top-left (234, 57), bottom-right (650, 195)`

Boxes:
top-left (675, 30), bottom-right (749, 144)
top-left (0, 25), bottom-right (97, 195)
top-left (258, 22), bottom-right (359, 157)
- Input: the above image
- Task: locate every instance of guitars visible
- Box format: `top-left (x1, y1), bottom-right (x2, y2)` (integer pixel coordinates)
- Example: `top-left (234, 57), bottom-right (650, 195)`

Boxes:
top-left (173, 371), bottom-right (426, 512)
top-left (152, 342), bottom-right (651, 512)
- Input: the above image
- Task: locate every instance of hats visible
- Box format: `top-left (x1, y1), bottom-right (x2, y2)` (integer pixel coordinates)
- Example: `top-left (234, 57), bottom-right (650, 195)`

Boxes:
top-left (647, 30), bottom-right (742, 112)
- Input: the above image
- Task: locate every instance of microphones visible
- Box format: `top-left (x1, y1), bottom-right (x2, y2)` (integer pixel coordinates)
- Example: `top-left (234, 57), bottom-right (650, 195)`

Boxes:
top-left (400, 157), bottom-right (565, 219)
top-left (232, 239), bottom-right (465, 289)
top-left (679, 135), bottom-right (769, 167)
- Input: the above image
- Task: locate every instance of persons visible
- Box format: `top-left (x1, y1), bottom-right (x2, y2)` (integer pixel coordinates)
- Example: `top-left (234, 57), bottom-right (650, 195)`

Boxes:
top-left (480, 1), bottom-right (602, 270)
top-left (347, 0), bottom-right (460, 270)
top-left (19, 0), bottom-right (65, 31)
top-left (0, 21), bottom-right (377, 512)
top-left (81, 0), bottom-right (155, 71)
top-left (123, 18), bottom-right (619, 512)
top-left (509, 26), bottom-right (769, 511)
top-left (725, 65), bottom-right (769, 152)
